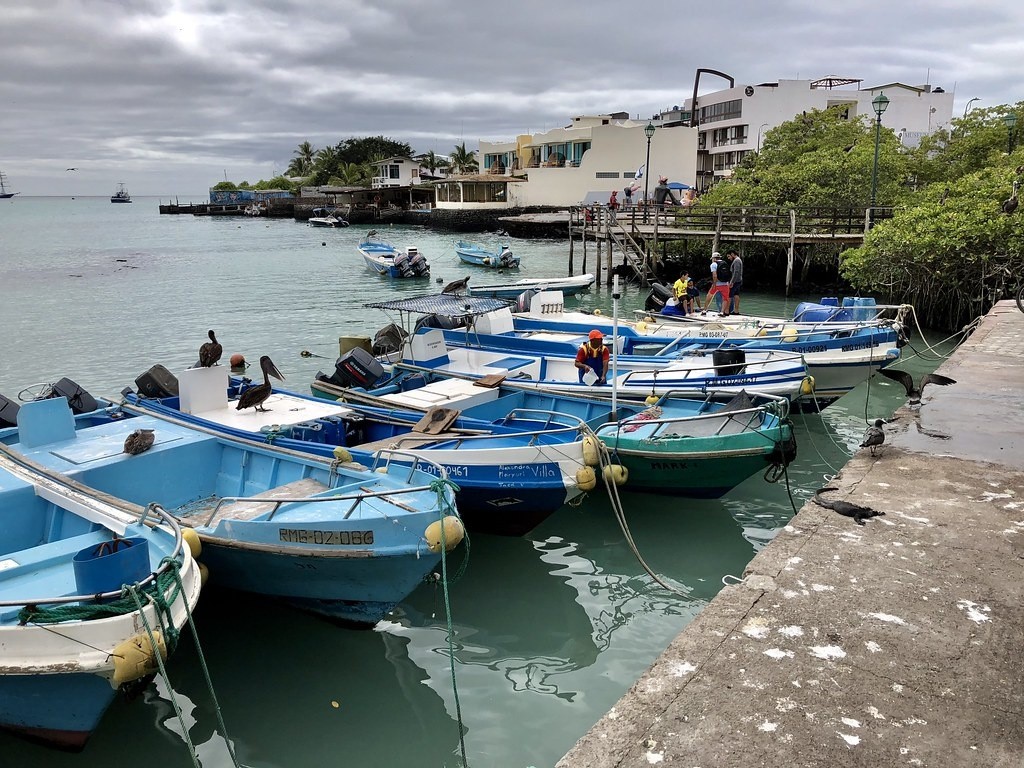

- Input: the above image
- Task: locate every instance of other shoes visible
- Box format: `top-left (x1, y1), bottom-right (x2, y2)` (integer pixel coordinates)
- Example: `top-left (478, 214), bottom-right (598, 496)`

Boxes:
top-left (723, 312), bottom-right (729, 316)
top-left (729, 311), bottom-right (740, 315)
top-left (701, 312), bottom-right (706, 315)
top-left (685, 313), bottom-right (690, 317)
top-left (610, 224), bottom-right (618, 227)
top-left (718, 313), bottom-right (725, 317)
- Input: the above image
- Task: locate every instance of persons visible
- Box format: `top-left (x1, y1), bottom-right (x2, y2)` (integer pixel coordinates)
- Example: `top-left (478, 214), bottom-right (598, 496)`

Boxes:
top-left (575, 330), bottom-right (610, 383)
top-left (623, 182), bottom-right (641, 202)
top-left (724, 250), bottom-right (743, 315)
top-left (657, 175), bottom-right (668, 185)
top-left (687, 277), bottom-right (701, 311)
top-left (701, 253), bottom-right (730, 315)
top-left (610, 191), bottom-right (619, 226)
top-left (673, 270), bottom-right (693, 316)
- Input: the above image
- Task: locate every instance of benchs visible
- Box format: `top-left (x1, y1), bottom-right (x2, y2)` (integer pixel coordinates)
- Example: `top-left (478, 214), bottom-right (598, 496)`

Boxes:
top-left (484, 357), bottom-right (537, 379)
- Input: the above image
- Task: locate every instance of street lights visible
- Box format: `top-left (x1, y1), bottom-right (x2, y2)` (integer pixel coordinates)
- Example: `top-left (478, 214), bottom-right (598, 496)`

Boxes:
top-left (869, 90), bottom-right (891, 231)
top-left (642, 121), bottom-right (656, 225)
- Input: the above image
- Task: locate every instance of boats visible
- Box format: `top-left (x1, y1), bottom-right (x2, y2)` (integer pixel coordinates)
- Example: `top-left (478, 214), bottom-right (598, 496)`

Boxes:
top-left (0, 376), bottom-right (465, 632)
top-left (453, 239), bottom-right (521, 269)
top-left (356, 235), bottom-right (431, 279)
top-left (466, 272), bottom-right (596, 298)
top-left (308, 207), bottom-right (350, 228)
top-left (309, 295), bottom-right (911, 501)
top-left (0, 453), bottom-right (209, 755)
top-left (118, 357), bottom-right (596, 537)
top-left (109, 183), bottom-right (133, 203)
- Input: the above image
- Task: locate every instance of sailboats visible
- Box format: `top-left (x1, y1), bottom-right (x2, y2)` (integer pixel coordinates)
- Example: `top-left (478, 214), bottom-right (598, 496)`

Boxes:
top-left (0, 169), bottom-right (20, 198)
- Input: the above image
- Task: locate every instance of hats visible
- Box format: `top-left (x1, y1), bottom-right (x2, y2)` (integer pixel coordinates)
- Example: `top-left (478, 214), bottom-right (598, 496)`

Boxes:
top-left (687, 277), bottom-right (692, 282)
top-left (589, 330), bottom-right (605, 340)
top-left (612, 191), bottom-right (617, 195)
top-left (711, 252), bottom-right (723, 258)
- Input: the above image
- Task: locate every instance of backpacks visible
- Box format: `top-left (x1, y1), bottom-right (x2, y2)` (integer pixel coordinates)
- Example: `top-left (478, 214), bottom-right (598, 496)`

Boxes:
top-left (714, 260), bottom-right (732, 282)
